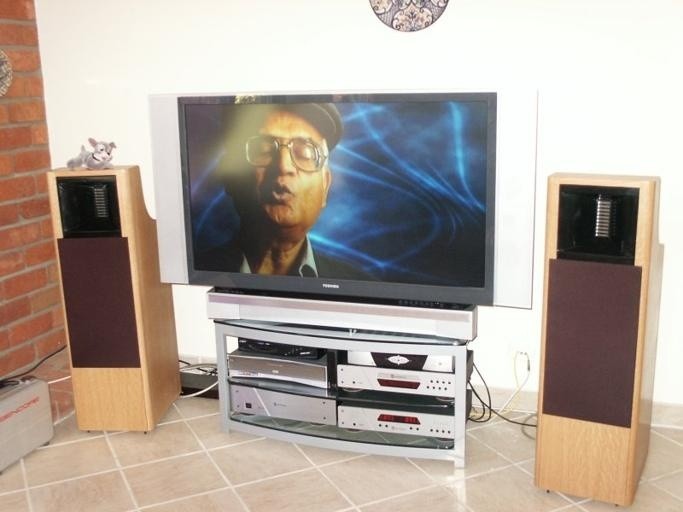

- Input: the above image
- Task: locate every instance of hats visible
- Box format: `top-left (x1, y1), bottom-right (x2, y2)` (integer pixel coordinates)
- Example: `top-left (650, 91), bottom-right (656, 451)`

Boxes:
top-left (221, 101), bottom-right (344, 150)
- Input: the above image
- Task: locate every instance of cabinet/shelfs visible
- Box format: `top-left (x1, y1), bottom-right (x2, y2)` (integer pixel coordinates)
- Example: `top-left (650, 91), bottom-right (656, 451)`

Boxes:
top-left (214, 322), bottom-right (470, 469)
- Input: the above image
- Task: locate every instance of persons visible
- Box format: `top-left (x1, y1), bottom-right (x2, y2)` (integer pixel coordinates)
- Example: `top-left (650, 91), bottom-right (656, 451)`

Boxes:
top-left (215, 103), bottom-right (375, 280)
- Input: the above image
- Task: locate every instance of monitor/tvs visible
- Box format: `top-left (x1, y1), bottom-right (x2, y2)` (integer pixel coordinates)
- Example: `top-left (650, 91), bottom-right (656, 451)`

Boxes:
top-left (178, 92), bottom-right (498, 309)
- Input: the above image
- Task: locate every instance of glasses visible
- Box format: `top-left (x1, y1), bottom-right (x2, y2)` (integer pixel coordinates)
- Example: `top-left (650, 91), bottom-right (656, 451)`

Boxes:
top-left (243, 136), bottom-right (329, 173)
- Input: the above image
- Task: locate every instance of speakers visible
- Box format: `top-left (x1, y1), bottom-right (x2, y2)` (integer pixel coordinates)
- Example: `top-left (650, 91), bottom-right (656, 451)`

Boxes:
top-left (534, 172), bottom-right (664, 507)
top-left (493, 91), bottom-right (539, 310)
top-left (46, 165), bottom-right (182, 432)
top-left (149, 93), bottom-right (189, 286)
